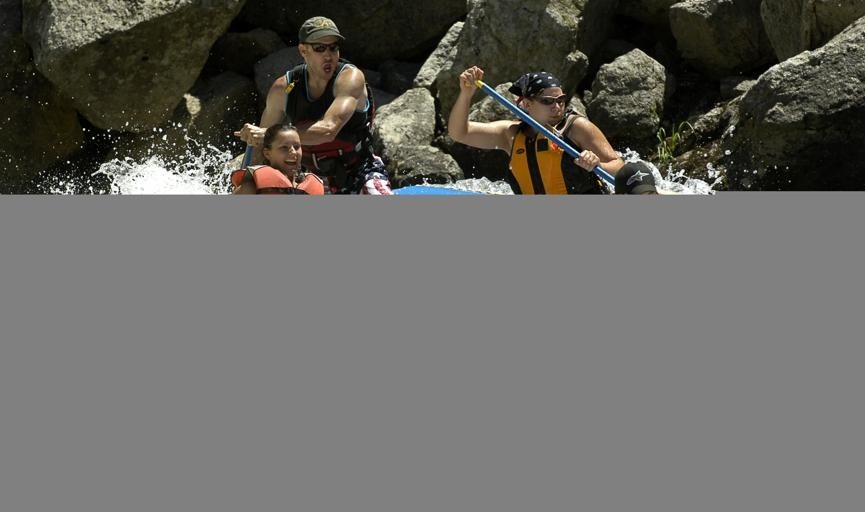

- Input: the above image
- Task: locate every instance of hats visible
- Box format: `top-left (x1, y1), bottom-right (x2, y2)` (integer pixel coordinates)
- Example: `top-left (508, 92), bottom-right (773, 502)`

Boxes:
top-left (615, 161), bottom-right (656, 194)
top-left (298, 16), bottom-right (346, 44)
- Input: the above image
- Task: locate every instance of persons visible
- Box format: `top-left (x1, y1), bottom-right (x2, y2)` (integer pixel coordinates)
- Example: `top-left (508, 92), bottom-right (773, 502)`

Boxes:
top-left (614, 159), bottom-right (658, 195)
top-left (447, 64), bottom-right (625, 195)
top-left (233, 16), bottom-right (394, 194)
top-left (230, 122), bottom-right (326, 195)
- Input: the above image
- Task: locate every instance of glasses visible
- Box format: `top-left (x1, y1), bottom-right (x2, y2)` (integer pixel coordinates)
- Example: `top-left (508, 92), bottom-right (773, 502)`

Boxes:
top-left (527, 94), bottom-right (568, 105)
top-left (301, 42), bottom-right (340, 52)
